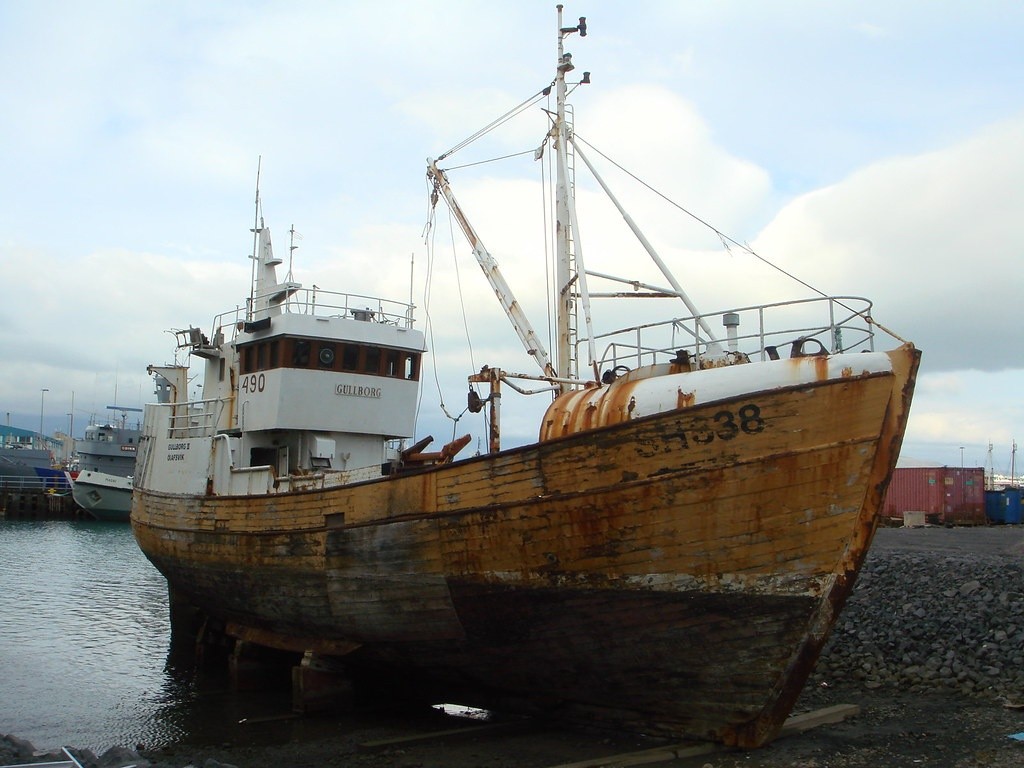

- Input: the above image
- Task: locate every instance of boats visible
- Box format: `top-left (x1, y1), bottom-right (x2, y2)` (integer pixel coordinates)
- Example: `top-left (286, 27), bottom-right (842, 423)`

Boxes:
top-left (0, 404), bottom-right (139, 520)
top-left (131, 4), bottom-right (923, 754)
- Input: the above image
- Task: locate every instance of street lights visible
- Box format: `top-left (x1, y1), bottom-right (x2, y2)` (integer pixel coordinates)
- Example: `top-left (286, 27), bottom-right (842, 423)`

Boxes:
top-left (39, 388), bottom-right (49, 434)
top-left (66, 413), bottom-right (73, 438)
top-left (959, 446), bottom-right (965, 468)
top-left (1011, 439), bottom-right (1018, 486)
